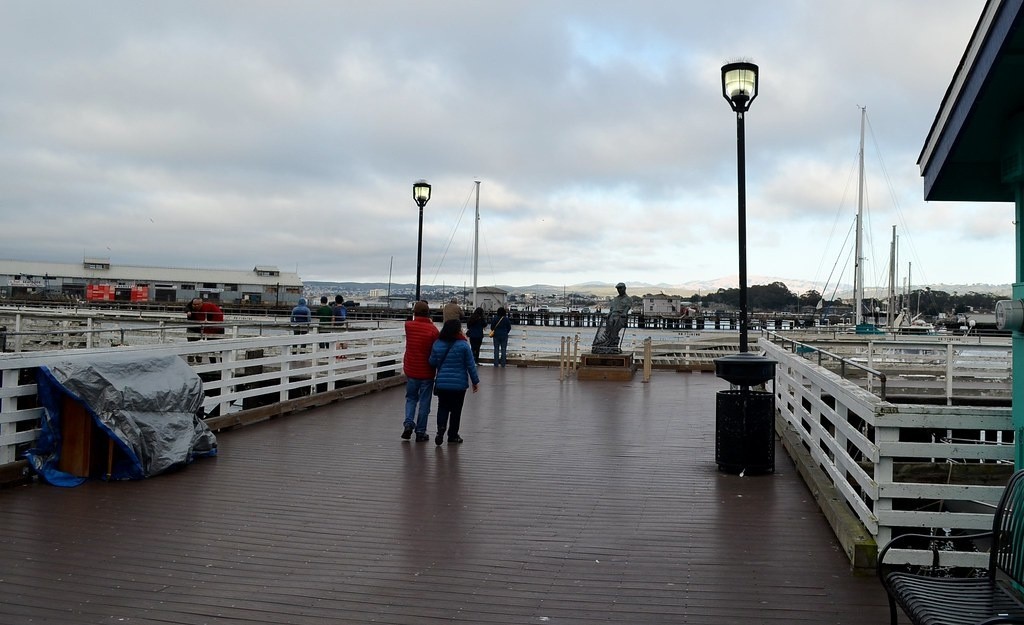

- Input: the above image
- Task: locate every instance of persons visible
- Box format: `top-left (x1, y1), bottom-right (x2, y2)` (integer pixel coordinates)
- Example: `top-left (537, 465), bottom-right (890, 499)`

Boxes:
top-left (442, 297), bottom-right (511, 368)
top-left (605, 283), bottom-right (633, 347)
top-left (400, 301), bottom-right (440, 443)
top-left (428, 319), bottom-right (480, 445)
top-left (185, 298), bottom-right (224, 364)
top-left (290, 294), bottom-right (348, 355)
top-left (680, 305), bottom-right (699, 328)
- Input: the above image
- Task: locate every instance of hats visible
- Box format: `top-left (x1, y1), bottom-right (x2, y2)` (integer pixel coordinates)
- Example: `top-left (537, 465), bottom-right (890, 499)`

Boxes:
top-left (413, 302), bottom-right (429, 317)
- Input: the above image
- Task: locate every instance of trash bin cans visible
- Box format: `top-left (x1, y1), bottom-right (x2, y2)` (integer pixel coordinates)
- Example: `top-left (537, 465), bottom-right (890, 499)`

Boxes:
top-left (714, 353), bottom-right (776, 476)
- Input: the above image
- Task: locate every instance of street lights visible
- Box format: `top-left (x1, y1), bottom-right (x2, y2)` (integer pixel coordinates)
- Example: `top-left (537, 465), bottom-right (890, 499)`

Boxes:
top-left (411, 179), bottom-right (432, 302)
top-left (719, 55), bottom-right (760, 352)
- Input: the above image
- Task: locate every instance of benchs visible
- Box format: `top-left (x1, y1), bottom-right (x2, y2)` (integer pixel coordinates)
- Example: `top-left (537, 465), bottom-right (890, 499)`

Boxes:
top-left (876, 469), bottom-right (1023, 624)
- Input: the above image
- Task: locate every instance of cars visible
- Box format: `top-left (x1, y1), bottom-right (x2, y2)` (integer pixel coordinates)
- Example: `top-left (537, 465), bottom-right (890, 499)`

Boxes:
top-left (581, 307), bottom-right (591, 315)
top-left (571, 307), bottom-right (579, 313)
top-left (328, 300), bottom-right (360, 307)
top-left (510, 304), bottom-right (518, 311)
top-left (537, 304), bottom-right (550, 312)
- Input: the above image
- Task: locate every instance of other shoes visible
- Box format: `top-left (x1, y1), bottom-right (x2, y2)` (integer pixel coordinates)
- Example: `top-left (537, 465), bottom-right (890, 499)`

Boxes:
top-left (416, 432), bottom-right (429, 442)
top-left (435, 432), bottom-right (444, 445)
top-left (400, 427), bottom-right (413, 439)
top-left (447, 434), bottom-right (464, 445)
top-left (475, 362), bottom-right (483, 366)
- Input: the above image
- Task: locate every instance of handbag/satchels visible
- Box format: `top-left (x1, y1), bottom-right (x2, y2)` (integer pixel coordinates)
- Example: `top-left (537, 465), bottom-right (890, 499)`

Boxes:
top-left (432, 369), bottom-right (438, 396)
top-left (466, 329), bottom-right (471, 338)
top-left (489, 330), bottom-right (495, 338)
